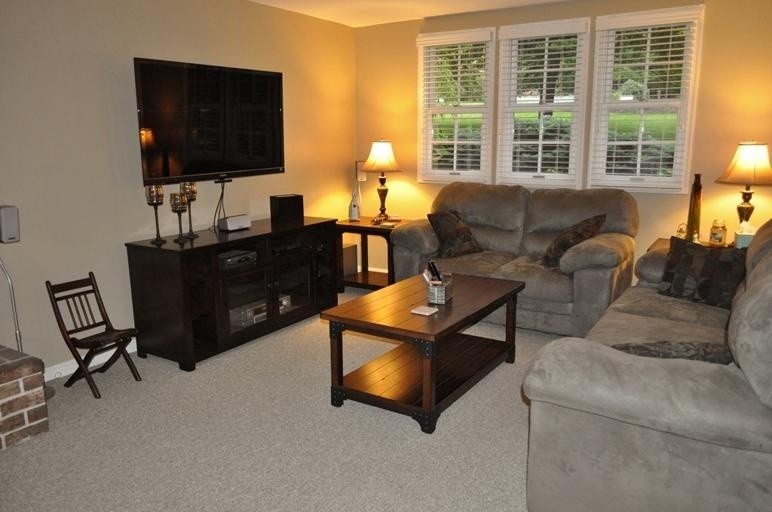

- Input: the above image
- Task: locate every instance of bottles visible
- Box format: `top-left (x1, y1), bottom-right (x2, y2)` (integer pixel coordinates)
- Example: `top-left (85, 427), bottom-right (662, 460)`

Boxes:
top-left (349, 189), bottom-right (361, 221)
top-left (709, 219), bottom-right (727, 247)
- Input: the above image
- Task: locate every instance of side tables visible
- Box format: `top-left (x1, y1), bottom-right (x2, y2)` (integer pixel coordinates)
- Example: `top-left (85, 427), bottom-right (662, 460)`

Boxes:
top-left (336, 216), bottom-right (398, 290)
top-left (647, 237), bottom-right (730, 256)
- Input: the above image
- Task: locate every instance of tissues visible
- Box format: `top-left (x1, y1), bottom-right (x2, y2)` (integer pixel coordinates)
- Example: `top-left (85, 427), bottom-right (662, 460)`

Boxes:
top-left (735, 220), bottom-right (756, 250)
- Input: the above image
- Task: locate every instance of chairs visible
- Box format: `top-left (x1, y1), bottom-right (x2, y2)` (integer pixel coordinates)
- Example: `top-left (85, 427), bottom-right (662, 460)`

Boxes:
top-left (46, 270), bottom-right (141, 398)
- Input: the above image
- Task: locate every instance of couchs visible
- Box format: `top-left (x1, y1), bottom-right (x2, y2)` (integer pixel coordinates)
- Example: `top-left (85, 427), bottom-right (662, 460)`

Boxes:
top-left (522, 219), bottom-right (772, 512)
top-left (390, 182), bottom-right (639, 338)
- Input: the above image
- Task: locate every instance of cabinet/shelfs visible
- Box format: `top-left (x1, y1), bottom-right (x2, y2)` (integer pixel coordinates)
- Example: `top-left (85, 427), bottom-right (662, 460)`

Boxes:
top-left (124, 212), bottom-right (339, 372)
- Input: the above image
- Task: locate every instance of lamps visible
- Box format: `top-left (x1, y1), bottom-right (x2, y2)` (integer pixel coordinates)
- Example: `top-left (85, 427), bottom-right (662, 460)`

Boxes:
top-left (361, 141), bottom-right (404, 225)
top-left (0, 204), bottom-right (55, 401)
top-left (712, 141), bottom-right (771, 250)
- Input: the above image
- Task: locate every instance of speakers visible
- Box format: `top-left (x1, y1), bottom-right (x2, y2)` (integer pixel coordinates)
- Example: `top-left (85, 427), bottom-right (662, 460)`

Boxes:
top-left (1, 205), bottom-right (20, 242)
top-left (356, 161), bottom-right (366, 182)
top-left (270, 193), bottom-right (304, 230)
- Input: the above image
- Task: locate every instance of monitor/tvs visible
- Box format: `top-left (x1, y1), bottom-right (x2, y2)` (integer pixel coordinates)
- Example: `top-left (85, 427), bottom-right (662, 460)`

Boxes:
top-left (133, 55), bottom-right (286, 185)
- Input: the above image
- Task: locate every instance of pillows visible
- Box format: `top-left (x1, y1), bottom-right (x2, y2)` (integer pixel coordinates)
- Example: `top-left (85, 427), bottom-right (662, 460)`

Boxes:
top-left (427, 209), bottom-right (484, 258)
top-left (541, 213), bottom-right (606, 266)
top-left (610, 340), bottom-right (731, 364)
top-left (659, 237), bottom-right (746, 310)
